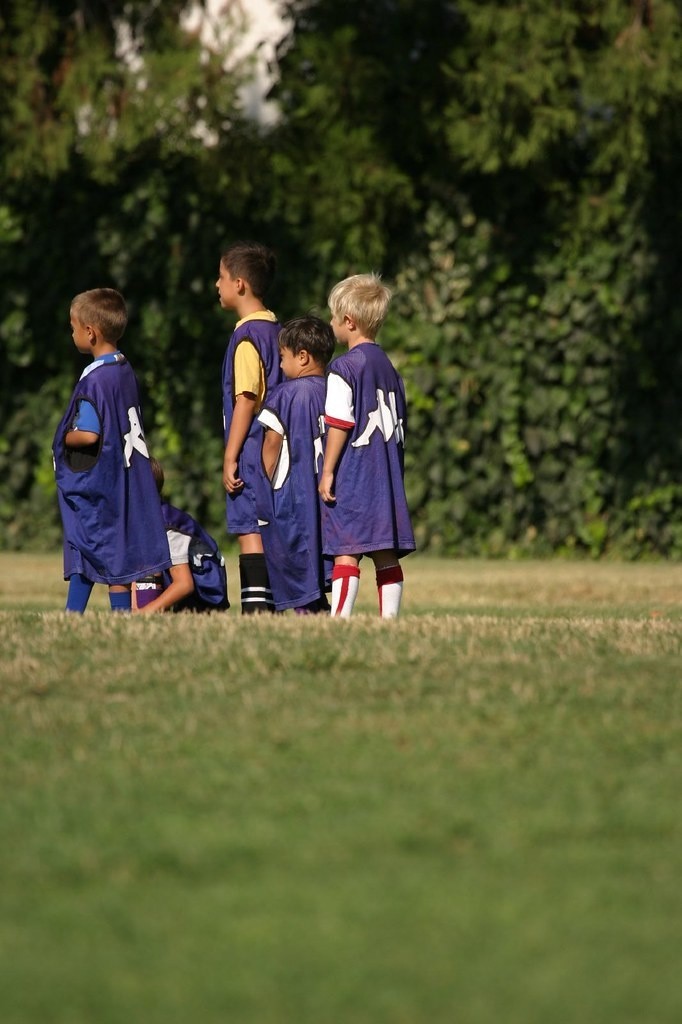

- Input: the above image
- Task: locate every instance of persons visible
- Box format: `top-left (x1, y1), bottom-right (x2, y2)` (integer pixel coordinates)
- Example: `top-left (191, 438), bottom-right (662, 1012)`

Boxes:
top-left (51, 288), bottom-right (171, 611)
top-left (317, 273), bottom-right (416, 619)
top-left (256, 316), bottom-right (337, 611)
top-left (131, 457), bottom-right (230, 614)
top-left (216, 246), bottom-right (283, 616)
